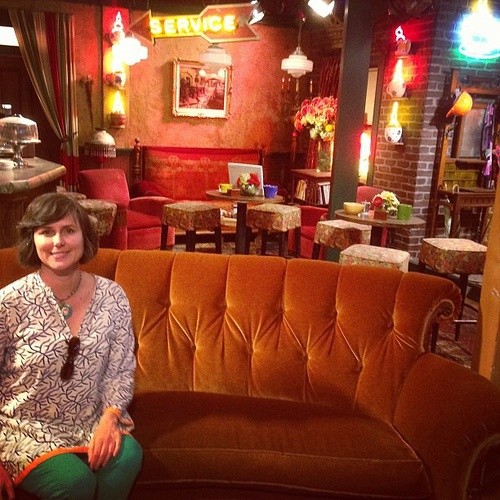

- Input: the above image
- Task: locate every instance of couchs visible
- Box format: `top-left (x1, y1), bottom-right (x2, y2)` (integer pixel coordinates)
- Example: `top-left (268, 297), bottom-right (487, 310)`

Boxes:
top-left (133, 138), bottom-right (269, 235)
top-left (0, 243), bottom-right (500, 500)
top-left (77, 167), bottom-right (178, 250)
top-left (288, 204), bottom-right (329, 257)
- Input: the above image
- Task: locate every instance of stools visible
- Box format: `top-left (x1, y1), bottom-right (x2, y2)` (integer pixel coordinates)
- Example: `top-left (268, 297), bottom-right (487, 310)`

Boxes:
top-left (338, 245), bottom-right (411, 272)
top-left (244, 202), bottom-right (302, 258)
top-left (159, 201), bottom-right (225, 255)
top-left (419, 236), bottom-right (488, 350)
top-left (308, 217), bottom-right (375, 263)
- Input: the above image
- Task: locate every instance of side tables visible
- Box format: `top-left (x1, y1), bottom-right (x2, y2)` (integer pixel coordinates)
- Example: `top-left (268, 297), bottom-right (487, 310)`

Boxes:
top-left (290, 167), bottom-right (331, 208)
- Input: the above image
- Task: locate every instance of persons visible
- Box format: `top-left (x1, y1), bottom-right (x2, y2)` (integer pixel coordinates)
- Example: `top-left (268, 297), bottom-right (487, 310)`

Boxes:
top-left (0, 192), bottom-right (142, 500)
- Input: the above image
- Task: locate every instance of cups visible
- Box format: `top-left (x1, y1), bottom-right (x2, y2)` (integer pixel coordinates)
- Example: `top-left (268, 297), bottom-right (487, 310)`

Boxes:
top-left (219, 184), bottom-right (233, 193)
top-left (398, 204), bottom-right (413, 220)
top-left (358, 201), bottom-right (372, 218)
top-left (0, 159), bottom-right (18, 171)
top-left (263, 185), bottom-right (278, 198)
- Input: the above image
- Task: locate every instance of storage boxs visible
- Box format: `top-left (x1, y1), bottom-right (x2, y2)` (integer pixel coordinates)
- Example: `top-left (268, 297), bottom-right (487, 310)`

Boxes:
top-left (227, 189), bottom-right (241, 197)
top-left (374, 209), bottom-right (387, 220)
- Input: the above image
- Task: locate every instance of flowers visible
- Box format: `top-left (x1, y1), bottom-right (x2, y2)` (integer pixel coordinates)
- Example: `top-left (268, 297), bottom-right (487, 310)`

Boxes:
top-left (295, 94), bottom-right (338, 139)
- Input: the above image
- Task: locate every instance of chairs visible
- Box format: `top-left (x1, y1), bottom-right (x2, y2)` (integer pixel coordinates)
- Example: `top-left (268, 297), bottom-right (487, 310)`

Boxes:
top-left (356, 185), bottom-right (394, 246)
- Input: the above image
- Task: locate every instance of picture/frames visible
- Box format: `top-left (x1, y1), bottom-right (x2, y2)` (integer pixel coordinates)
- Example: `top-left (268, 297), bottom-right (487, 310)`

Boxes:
top-left (171, 57), bottom-right (233, 121)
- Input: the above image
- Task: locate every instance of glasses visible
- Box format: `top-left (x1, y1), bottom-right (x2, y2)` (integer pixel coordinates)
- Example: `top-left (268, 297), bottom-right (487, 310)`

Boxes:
top-left (60, 336), bottom-right (81, 381)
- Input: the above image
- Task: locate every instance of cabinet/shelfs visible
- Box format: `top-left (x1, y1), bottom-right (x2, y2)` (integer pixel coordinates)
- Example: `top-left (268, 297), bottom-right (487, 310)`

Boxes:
top-left (427, 71), bottom-right (500, 240)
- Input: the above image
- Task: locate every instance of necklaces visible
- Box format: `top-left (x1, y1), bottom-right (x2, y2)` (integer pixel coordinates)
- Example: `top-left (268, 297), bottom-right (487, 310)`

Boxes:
top-left (54, 273), bottom-right (82, 320)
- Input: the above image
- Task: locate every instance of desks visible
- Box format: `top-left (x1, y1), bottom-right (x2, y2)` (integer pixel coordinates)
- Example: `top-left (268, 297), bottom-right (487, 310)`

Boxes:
top-left (334, 209), bottom-right (427, 249)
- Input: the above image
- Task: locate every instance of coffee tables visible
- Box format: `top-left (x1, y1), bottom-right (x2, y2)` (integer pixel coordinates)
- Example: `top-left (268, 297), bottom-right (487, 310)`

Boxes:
top-left (205, 182), bottom-right (285, 252)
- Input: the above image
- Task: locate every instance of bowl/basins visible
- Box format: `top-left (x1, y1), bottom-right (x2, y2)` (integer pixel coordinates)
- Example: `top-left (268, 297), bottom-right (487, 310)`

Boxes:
top-left (343, 202), bottom-right (363, 214)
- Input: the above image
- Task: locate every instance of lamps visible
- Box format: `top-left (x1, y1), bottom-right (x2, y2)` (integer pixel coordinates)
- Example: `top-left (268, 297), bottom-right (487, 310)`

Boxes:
top-left (104, 10), bottom-right (128, 44)
top-left (84, 124), bottom-right (116, 169)
top-left (108, 90), bottom-right (129, 128)
top-left (306, 0), bottom-right (336, 19)
top-left (386, 59), bottom-right (406, 100)
top-left (109, 37), bottom-right (150, 68)
top-left (385, 101), bottom-right (405, 145)
top-left (106, 54), bottom-right (128, 87)
top-left (279, 18), bottom-right (315, 79)
top-left (198, 41), bottom-right (232, 67)
top-left (458, 0), bottom-right (500, 61)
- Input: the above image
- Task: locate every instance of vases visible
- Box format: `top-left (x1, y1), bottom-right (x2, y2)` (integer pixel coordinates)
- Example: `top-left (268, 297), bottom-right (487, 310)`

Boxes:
top-left (317, 138), bottom-right (331, 172)
